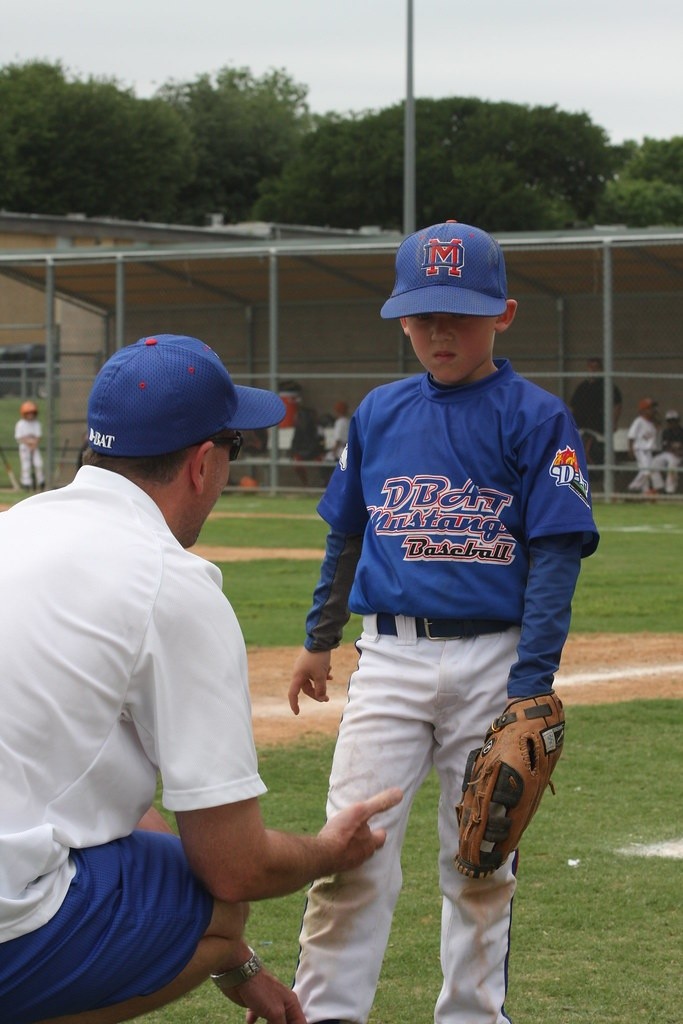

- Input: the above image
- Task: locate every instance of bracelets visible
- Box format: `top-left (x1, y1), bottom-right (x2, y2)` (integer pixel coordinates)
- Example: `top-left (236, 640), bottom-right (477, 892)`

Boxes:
top-left (209, 945), bottom-right (263, 990)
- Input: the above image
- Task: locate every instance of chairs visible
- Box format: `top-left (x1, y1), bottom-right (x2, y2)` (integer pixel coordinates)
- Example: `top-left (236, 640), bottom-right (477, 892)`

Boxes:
top-left (596, 428), bottom-right (631, 488)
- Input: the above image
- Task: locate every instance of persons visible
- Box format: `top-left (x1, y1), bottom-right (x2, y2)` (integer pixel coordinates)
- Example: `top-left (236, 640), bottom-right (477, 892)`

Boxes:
top-left (15, 399), bottom-right (46, 492)
top-left (0, 332), bottom-right (404, 1024)
top-left (289, 358), bottom-right (683, 501)
top-left (287, 219), bottom-right (600, 1023)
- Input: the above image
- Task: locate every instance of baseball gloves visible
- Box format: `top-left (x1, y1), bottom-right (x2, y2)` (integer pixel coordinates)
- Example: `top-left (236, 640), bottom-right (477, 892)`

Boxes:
top-left (453, 688), bottom-right (567, 880)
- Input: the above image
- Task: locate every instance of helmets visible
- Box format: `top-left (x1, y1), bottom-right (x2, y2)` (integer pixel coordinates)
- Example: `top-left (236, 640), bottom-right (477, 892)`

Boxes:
top-left (21, 403), bottom-right (38, 419)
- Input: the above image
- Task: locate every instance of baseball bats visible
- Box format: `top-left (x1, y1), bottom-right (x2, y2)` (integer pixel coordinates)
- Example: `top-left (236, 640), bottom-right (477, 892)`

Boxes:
top-left (0, 446), bottom-right (21, 489)
top-left (56, 438), bottom-right (70, 480)
top-left (30, 448), bottom-right (37, 490)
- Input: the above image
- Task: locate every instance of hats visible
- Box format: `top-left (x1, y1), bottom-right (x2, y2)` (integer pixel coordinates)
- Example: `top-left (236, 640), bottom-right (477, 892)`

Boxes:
top-left (639, 398), bottom-right (657, 410)
top-left (665, 410), bottom-right (679, 421)
top-left (380, 219), bottom-right (508, 319)
top-left (87, 332), bottom-right (287, 457)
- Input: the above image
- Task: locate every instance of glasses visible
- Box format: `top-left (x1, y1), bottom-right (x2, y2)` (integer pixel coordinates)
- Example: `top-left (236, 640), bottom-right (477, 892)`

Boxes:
top-left (189, 431), bottom-right (243, 461)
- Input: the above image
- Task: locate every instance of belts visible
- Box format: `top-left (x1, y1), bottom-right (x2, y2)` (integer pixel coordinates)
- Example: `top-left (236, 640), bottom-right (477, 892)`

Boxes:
top-left (375, 610), bottom-right (513, 641)
